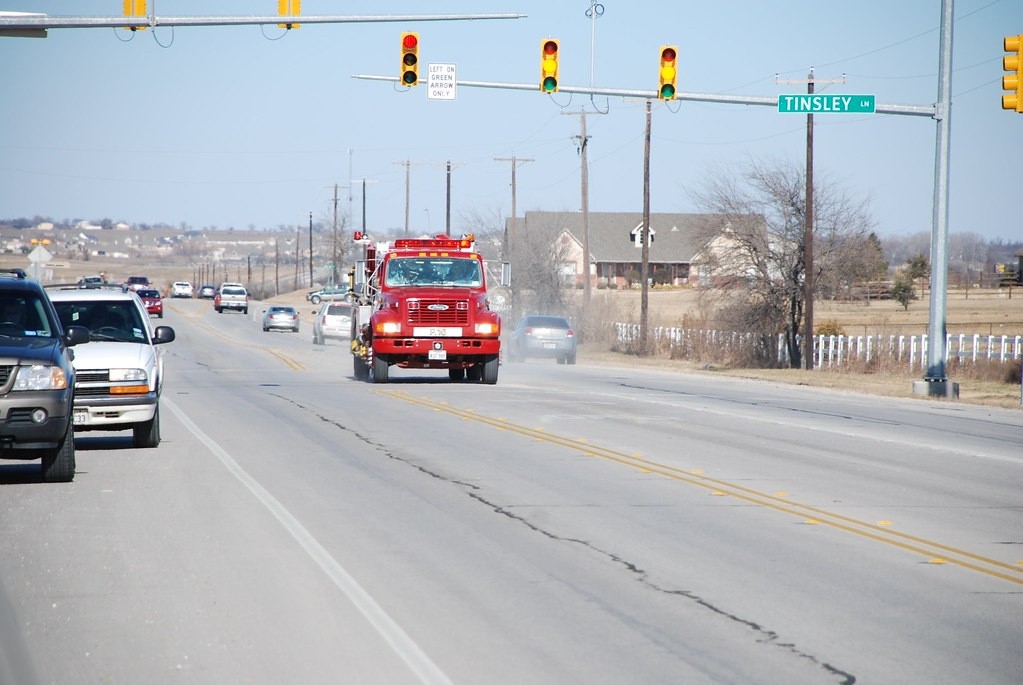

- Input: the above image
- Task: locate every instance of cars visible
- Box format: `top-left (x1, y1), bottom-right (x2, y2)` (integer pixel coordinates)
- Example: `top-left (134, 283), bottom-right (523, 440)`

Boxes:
top-left (170, 282), bottom-right (195, 299)
top-left (123, 276), bottom-right (153, 290)
top-left (80, 276), bottom-right (105, 286)
top-left (197, 285), bottom-right (216, 300)
top-left (507, 315), bottom-right (579, 367)
top-left (306, 284), bottom-right (353, 303)
top-left (133, 289), bottom-right (166, 318)
top-left (217, 282), bottom-right (248, 295)
top-left (309, 302), bottom-right (351, 346)
top-left (262, 305), bottom-right (299, 332)
top-left (215, 286), bottom-right (252, 315)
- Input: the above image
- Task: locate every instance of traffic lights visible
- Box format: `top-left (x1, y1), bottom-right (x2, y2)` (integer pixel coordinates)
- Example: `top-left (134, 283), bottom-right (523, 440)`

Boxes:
top-left (539, 37), bottom-right (559, 95)
top-left (400, 31), bottom-right (418, 88)
top-left (658, 45), bottom-right (678, 101)
top-left (1001, 34), bottom-right (1023, 114)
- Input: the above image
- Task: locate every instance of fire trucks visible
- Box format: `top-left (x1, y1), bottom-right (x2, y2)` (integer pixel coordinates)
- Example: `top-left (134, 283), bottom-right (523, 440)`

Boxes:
top-left (347, 230), bottom-right (502, 384)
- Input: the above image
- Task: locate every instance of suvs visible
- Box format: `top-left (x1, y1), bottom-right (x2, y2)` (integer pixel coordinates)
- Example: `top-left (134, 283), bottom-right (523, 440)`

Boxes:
top-left (38, 282), bottom-right (176, 448)
top-left (0, 267), bottom-right (80, 481)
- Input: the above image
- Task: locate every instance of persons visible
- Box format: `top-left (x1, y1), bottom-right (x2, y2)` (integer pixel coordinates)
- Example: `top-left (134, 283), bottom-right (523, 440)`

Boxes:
top-left (443, 261), bottom-right (466, 281)
top-left (414, 261), bottom-right (444, 283)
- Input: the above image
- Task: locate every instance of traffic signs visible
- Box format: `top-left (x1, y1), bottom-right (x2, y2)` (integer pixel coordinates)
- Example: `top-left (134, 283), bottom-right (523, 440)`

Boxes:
top-left (427, 62), bottom-right (456, 101)
top-left (777, 92), bottom-right (877, 115)
